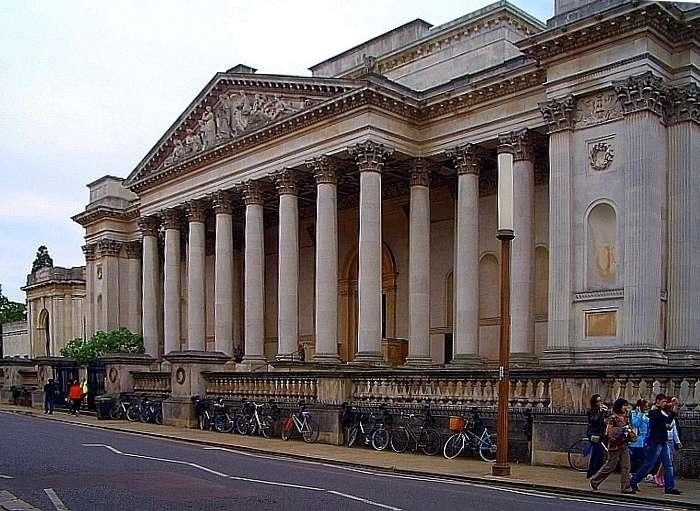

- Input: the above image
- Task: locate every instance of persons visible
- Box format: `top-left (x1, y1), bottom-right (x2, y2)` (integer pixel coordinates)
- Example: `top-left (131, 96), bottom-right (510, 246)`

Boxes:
top-left (232, 343), bottom-right (243, 363)
top-left (153, 89), bottom-right (318, 159)
top-left (298, 343), bottom-right (305, 362)
top-left (42, 378), bottom-right (57, 415)
top-left (195, 396), bottom-right (214, 430)
top-left (67, 383), bottom-right (82, 414)
top-left (584, 390), bottom-right (686, 495)
top-left (73, 374), bottom-right (89, 398)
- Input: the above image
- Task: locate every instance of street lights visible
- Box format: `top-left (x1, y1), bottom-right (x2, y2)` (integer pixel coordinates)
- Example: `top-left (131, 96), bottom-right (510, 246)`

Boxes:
top-left (489, 144), bottom-right (520, 476)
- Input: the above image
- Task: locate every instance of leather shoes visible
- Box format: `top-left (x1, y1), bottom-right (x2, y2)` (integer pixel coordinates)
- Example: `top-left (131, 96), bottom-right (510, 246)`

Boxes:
top-left (653, 475), bottom-right (665, 487)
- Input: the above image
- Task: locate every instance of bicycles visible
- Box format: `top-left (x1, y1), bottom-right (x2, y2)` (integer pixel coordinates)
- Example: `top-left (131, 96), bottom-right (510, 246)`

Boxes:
top-left (390, 410), bottom-right (441, 457)
top-left (347, 406), bottom-right (389, 452)
top-left (109, 392), bottom-right (169, 425)
top-left (567, 436), bottom-right (609, 473)
top-left (190, 393), bottom-right (320, 444)
top-left (442, 414), bottom-right (498, 463)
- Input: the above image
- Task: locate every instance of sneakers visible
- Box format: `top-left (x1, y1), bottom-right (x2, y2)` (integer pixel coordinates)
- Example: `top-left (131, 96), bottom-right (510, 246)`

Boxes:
top-left (589, 479), bottom-right (600, 491)
top-left (621, 483), bottom-right (640, 494)
top-left (665, 489), bottom-right (682, 494)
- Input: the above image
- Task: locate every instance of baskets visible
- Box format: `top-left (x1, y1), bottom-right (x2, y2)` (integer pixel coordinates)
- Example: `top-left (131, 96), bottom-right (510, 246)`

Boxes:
top-left (449, 416), bottom-right (464, 431)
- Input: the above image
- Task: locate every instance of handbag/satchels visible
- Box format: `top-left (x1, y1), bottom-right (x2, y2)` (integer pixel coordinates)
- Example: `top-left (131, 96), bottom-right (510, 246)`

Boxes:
top-left (591, 435), bottom-right (600, 443)
